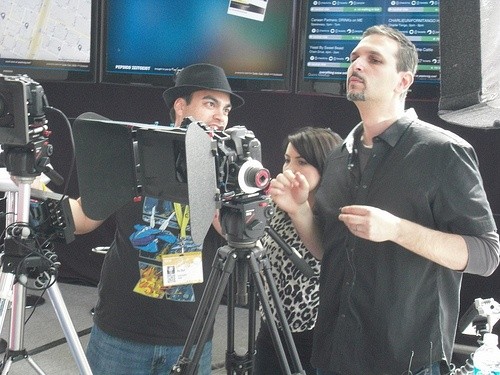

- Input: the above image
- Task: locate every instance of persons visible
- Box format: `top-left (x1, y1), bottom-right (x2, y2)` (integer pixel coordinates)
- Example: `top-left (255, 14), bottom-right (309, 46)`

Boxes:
top-left (31, 62), bottom-right (245, 375)
top-left (253, 126), bottom-right (345, 375)
top-left (268, 23), bottom-right (500, 375)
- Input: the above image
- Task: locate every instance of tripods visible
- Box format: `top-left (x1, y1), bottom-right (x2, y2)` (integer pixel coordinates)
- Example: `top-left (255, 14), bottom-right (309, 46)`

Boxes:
top-left (0, 137), bottom-right (95, 375)
top-left (170, 197), bottom-right (313, 375)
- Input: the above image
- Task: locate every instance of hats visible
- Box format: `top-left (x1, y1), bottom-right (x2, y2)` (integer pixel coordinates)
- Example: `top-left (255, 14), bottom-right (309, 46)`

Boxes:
top-left (162, 63), bottom-right (246, 111)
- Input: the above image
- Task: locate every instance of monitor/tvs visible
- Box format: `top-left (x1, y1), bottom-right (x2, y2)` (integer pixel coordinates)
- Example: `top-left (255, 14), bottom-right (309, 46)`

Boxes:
top-left (0, 0), bottom-right (483, 100)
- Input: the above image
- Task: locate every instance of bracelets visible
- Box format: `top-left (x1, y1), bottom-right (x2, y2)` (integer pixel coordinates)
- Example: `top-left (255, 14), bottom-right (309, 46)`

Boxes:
top-left (32, 181), bottom-right (46, 202)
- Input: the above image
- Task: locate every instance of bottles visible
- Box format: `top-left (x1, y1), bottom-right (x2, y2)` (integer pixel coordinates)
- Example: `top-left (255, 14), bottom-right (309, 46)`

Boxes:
top-left (473, 332), bottom-right (500, 375)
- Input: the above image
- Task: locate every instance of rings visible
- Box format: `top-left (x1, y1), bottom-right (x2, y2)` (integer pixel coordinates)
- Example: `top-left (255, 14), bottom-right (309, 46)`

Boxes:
top-left (356, 224), bottom-right (361, 232)
